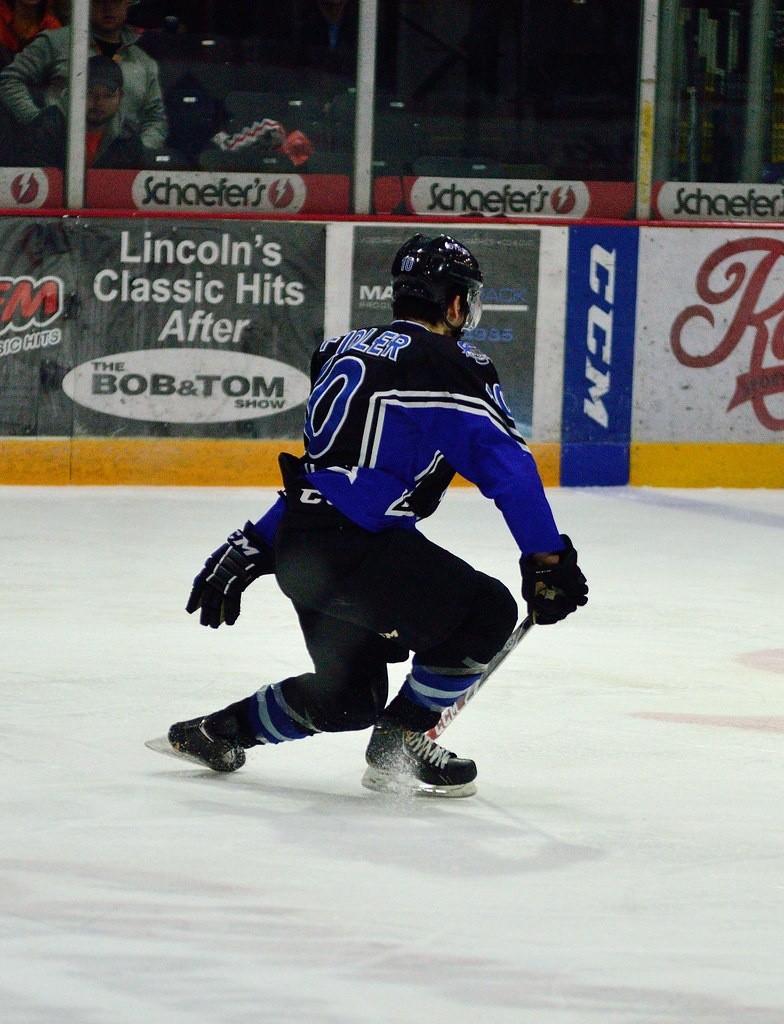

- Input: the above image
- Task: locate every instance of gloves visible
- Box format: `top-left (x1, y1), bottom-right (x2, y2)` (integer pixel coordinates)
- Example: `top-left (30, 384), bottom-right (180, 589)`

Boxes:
top-left (520, 534), bottom-right (588, 624)
top-left (187, 519), bottom-right (272, 629)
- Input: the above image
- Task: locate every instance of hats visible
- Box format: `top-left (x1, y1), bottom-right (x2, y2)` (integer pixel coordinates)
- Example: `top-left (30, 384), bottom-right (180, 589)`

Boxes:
top-left (88, 55), bottom-right (123, 92)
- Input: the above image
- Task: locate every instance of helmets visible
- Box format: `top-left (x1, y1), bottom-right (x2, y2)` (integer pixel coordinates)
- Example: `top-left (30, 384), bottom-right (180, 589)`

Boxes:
top-left (392, 233), bottom-right (484, 309)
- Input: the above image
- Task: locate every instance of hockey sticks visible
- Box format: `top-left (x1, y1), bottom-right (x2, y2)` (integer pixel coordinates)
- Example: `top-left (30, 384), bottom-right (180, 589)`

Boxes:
top-left (423, 603), bottom-right (540, 740)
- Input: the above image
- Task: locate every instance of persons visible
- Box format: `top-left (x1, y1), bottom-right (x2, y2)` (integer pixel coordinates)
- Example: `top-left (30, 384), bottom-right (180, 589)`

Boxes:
top-left (0, 0), bottom-right (313, 173)
top-left (143, 234), bottom-right (589, 798)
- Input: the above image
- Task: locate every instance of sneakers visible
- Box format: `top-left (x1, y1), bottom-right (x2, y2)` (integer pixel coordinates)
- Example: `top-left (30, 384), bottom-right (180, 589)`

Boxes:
top-left (361, 716), bottom-right (478, 798)
top-left (145, 716), bottom-right (246, 773)
